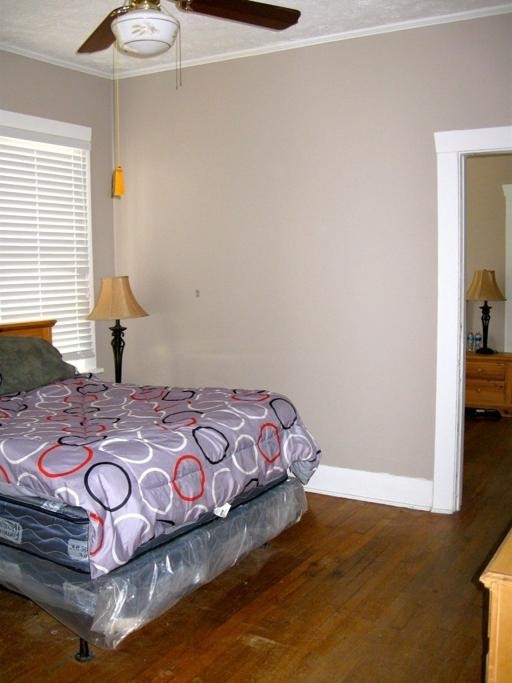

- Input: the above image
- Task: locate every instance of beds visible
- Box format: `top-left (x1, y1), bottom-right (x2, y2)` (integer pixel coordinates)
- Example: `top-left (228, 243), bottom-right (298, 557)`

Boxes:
top-left (0, 320), bottom-right (322, 663)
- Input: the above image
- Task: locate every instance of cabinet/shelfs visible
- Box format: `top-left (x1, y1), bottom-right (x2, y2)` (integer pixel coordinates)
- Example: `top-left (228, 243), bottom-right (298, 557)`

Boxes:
top-left (477, 528), bottom-right (512, 683)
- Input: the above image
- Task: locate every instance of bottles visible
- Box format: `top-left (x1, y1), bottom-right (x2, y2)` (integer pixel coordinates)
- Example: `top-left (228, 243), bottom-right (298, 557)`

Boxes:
top-left (467, 330), bottom-right (474, 353)
top-left (475, 330), bottom-right (482, 351)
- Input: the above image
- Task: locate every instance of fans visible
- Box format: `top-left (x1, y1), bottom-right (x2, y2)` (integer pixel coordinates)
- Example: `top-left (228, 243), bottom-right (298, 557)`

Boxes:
top-left (75, 0), bottom-right (302, 91)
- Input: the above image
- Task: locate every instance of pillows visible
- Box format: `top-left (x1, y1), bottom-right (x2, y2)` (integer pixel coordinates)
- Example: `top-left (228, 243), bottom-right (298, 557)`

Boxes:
top-left (0, 336), bottom-right (76, 397)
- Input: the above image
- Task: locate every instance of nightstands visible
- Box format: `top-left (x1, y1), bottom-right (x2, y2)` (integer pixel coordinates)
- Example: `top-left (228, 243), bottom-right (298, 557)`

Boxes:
top-left (465, 351), bottom-right (512, 418)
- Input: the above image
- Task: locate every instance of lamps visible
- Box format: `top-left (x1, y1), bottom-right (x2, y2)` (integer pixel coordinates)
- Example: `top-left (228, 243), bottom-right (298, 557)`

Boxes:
top-left (110, 9), bottom-right (181, 198)
top-left (86, 276), bottom-right (150, 384)
top-left (464, 270), bottom-right (508, 355)
